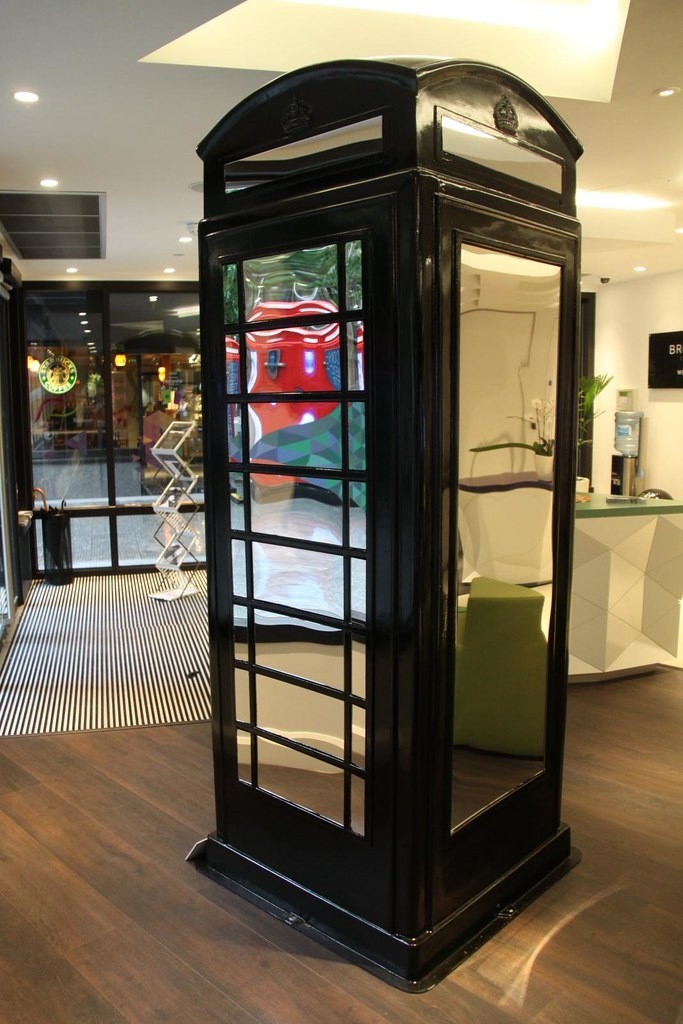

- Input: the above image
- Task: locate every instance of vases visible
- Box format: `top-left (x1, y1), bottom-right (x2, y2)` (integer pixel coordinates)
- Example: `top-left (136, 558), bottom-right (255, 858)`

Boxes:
top-left (533, 454), bottom-right (553, 481)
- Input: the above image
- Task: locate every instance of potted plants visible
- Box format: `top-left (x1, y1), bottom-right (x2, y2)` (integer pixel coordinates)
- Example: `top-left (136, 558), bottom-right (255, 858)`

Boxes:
top-left (575, 375), bottom-right (614, 493)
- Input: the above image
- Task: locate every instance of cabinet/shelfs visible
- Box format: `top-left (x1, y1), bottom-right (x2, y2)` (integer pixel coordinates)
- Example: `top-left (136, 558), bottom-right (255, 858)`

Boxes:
top-left (457, 474), bottom-right (555, 587)
top-left (151, 421), bottom-right (201, 602)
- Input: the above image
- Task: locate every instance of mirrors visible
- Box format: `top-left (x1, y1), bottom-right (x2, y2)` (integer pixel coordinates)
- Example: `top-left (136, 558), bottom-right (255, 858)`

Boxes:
top-left (451, 232), bottom-right (566, 834)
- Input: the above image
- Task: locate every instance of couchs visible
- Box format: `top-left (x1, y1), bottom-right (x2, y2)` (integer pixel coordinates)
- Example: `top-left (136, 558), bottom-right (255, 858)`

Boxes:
top-left (454, 577), bottom-right (548, 759)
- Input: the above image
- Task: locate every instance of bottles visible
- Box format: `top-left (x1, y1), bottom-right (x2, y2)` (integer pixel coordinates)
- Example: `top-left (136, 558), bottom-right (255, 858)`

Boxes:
top-left (614, 411), bottom-right (643, 456)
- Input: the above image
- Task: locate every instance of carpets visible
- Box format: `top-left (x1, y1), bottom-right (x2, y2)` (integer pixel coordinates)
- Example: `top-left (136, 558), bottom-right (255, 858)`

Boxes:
top-left (0, 567), bottom-right (212, 737)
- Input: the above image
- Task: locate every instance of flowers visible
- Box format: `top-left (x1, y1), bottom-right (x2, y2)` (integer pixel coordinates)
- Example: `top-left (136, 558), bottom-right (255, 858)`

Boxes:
top-left (469, 398), bottom-right (556, 457)
